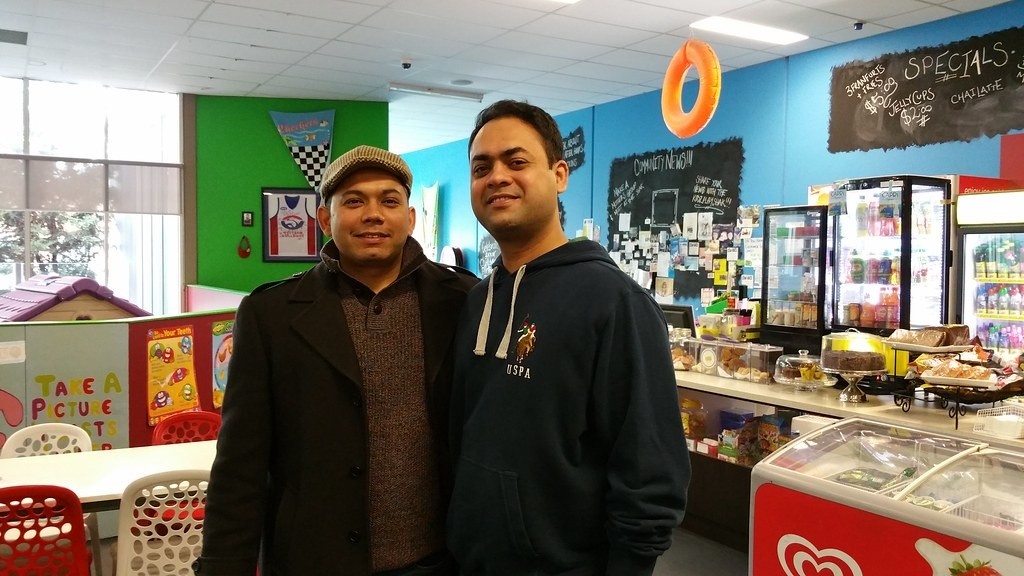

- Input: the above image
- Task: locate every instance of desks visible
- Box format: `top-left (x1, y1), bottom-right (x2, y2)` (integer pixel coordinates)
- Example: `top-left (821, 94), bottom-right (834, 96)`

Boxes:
top-left (0, 439), bottom-right (217, 524)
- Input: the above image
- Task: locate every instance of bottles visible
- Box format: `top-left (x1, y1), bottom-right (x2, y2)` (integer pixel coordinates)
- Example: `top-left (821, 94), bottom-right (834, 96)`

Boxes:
top-left (851, 247), bottom-right (930, 285)
top-left (976, 323), bottom-right (1024, 355)
top-left (974, 237), bottom-right (1024, 313)
top-left (861, 287), bottom-right (901, 329)
top-left (858, 191), bottom-right (931, 237)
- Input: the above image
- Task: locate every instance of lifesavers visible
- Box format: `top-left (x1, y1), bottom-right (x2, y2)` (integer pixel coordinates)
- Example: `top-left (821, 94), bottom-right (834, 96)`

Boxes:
top-left (660, 38), bottom-right (722, 140)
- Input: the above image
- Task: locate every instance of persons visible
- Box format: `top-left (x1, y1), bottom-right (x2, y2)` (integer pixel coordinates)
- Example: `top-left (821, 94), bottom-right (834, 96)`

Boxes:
top-left (191, 145), bottom-right (486, 576)
top-left (446, 101), bottom-right (691, 576)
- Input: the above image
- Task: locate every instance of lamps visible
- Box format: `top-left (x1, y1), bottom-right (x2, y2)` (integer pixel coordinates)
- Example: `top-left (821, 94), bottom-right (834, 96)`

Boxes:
top-left (388, 82), bottom-right (483, 103)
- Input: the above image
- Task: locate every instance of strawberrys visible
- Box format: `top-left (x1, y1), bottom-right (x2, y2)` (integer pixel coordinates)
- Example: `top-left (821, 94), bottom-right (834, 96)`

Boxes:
top-left (949, 554), bottom-right (1002, 576)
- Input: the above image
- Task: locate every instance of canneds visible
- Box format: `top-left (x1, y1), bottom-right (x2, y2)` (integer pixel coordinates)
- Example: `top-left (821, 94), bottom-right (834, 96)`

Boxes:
top-left (851, 257), bottom-right (892, 285)
top-left (843, 302), bottom-right (860, 327)
top-left (843, 360), bottom-right (844, 361)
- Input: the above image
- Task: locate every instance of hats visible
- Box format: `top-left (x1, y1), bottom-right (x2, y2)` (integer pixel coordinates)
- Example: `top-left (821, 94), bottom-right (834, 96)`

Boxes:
top-left (321, 145), bottom-right (413, 205)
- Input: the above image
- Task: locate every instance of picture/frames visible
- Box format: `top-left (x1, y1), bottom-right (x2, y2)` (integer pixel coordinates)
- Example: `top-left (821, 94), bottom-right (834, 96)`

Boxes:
top-left (261, 186), bottom-right (324, 262)
top-left (241, 212), bottom-right (254, 226)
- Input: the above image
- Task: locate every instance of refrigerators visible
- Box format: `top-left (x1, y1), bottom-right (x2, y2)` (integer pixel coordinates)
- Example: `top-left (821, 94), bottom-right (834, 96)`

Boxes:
top-left (832, 175), bottom-right (1017, 337)
top-left (759, 205), bottom-right (835, 358)
top-left (956, 189), bottom-right (1024, 359)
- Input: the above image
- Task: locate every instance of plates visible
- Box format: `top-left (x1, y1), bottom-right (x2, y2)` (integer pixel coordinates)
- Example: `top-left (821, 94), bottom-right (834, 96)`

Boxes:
top-left (919, 369), bottom-right (997, 387)
top-left (774, 378), bottom-right (838, 390)
top-left (887, 342), bottom-right (975, 352)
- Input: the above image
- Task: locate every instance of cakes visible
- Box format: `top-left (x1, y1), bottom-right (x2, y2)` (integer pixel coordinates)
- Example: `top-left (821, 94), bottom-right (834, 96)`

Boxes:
top-left (781, 349), bottom-right (887, 380)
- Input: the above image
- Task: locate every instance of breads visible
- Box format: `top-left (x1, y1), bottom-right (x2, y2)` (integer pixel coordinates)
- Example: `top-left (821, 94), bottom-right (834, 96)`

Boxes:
top-left (887, 323), bottom-right (992, 380)
top-left (721, 347), bottom-right (769, 380)
top-left (670, 346), bottom-right (694, 369)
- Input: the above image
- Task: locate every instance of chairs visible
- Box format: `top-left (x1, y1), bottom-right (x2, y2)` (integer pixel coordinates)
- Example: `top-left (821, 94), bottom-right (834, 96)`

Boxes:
top-left (0, 485), bottom-right (89, 576)
top-left (116, 469), bottom-right (262, 576)
top-left (0, 422), bottom-right (103, 576)
top-left (152, 412), bottom-right (223, 526)
